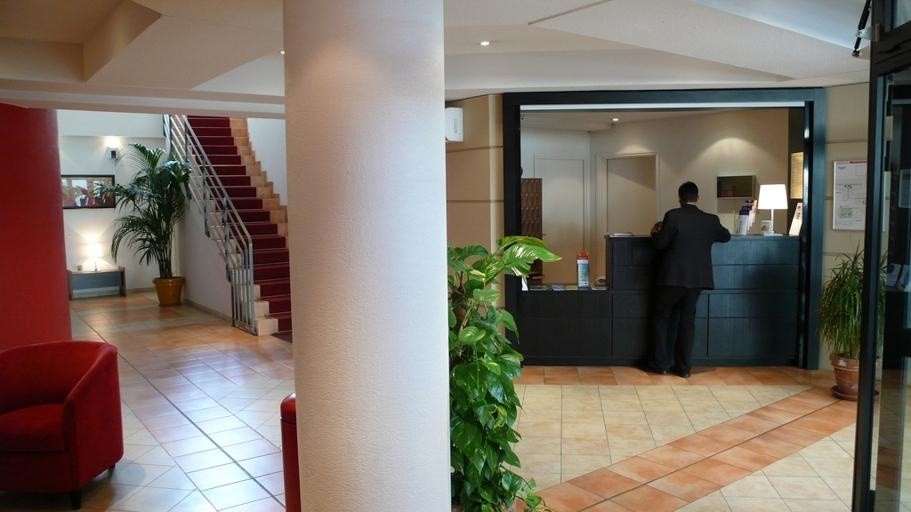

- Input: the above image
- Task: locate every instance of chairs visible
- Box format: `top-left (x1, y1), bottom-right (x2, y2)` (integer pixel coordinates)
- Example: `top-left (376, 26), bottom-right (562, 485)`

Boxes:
top-left (0, 339), bottom-right (124, 510)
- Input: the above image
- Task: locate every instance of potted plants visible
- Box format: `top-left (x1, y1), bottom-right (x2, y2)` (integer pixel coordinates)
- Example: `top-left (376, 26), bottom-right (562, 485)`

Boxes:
top-left (87, 142), bottom-right (194, 307)
top-left (816, 239), bottom-right (887, 400)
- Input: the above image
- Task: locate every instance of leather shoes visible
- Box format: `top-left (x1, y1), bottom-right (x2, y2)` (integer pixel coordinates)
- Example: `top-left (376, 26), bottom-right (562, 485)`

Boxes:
top-left (640, 366), bottom-right (689, 378)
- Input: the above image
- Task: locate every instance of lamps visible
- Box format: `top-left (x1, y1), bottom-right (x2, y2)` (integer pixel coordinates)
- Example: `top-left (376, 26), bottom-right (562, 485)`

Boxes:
top-left (104, 136), bottom-right (121, 161)
top-left (757, 183), bottom-right (789, 237)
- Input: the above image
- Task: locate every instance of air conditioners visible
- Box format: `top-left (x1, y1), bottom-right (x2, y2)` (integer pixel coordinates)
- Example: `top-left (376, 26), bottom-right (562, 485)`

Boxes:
top-left (444, 107), bottom-right (465, 142)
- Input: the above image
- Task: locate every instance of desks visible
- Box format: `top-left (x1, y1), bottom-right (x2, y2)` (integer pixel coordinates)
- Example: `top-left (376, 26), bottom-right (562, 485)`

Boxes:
top-left (66, 265), bottom-right (126, 300)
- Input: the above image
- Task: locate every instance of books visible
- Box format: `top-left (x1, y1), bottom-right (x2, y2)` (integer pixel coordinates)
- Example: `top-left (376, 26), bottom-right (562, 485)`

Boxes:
top-left (608, 232), bottom-right (632, 237)
top-left (737, 199), bottom-right (758, 234)
top-left (529, 284), bottom-right (547, 291)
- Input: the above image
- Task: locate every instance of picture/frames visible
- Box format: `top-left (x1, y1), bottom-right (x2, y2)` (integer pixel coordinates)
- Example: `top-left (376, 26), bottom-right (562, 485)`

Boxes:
top-left (60, 174), bottom-right (117, 209)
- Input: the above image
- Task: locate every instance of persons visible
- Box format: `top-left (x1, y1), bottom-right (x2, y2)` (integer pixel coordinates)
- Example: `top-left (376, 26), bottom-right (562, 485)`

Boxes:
top-left (642, 181), bottom-right (731, 377)
